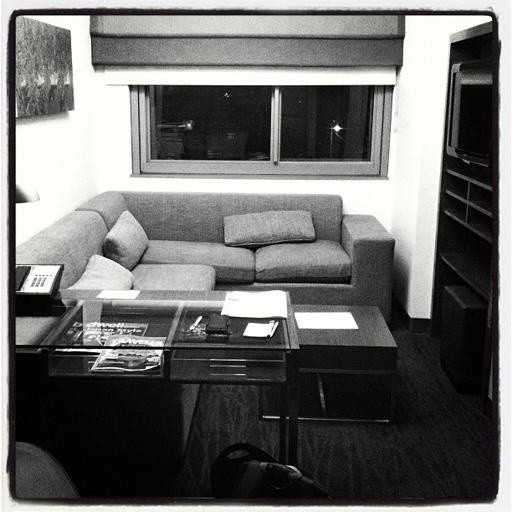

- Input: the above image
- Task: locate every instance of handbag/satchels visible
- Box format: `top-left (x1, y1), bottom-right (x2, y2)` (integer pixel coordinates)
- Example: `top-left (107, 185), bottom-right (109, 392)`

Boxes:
top-left (230, 460), bottom-right (328, 499)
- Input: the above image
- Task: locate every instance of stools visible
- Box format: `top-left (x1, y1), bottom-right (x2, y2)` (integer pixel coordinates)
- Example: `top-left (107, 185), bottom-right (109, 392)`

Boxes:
top-left (15, 297), bottom-right (78, 421)
top-left (260, 301), bottom-right (398, 422)
top-left (15, 444), bottom-right (80, 500)
top-left (439, 287), bottom-right (490, 373)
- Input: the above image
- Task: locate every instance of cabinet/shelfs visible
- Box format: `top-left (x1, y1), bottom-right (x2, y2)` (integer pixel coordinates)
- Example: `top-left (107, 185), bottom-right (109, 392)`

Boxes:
top-left (431, 21), bottom-right (500, 424)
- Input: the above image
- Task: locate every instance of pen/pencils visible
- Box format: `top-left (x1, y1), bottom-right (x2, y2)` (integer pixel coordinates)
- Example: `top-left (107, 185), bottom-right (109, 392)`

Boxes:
top-left (267, 320), bottom-right (275, 338)
top-left (190, 316), bottom-right (202, 331)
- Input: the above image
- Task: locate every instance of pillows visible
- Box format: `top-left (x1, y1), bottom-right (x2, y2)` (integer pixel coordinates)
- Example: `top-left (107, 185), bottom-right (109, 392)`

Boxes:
top-left (103, 212), bottom-right (150, 270)
top-left (224, 210), bottom-right (315, 249)
top-left (68, 254), bottom-right (134, 290)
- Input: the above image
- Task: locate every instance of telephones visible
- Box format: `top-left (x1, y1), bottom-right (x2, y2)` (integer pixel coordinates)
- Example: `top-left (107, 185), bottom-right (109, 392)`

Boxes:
top-left (15, 264), bottom-right (64, 299)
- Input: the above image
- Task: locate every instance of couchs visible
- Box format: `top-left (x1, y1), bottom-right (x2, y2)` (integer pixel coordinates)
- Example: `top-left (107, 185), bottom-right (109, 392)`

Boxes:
top-left (16, 191), bottom-right (395, 324)
